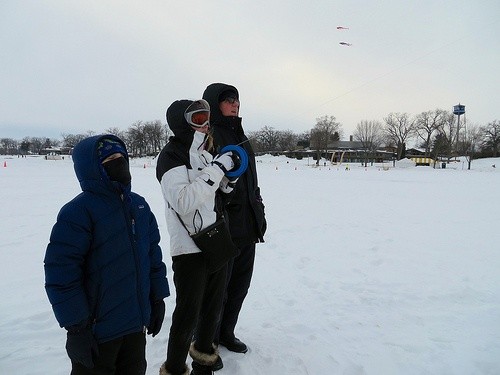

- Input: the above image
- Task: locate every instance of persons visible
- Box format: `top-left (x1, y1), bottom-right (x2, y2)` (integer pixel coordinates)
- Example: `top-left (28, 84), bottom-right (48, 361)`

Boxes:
top-left (43, 134), bottom-right (170, 375)
top-left (202, 83), bottom-right (267, 372)
top-left (156, 99), bottom-right (240, 375)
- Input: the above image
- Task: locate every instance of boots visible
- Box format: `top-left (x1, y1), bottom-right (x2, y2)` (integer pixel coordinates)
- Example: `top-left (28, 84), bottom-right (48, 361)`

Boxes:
top-left (189, 339), bottom-right (220, 375)
top-left (158, 360), bottom-right (190, 375)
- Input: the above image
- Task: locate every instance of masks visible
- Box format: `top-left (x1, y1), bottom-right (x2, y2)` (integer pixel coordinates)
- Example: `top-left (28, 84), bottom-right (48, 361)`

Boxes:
top-left (102, 156), bottom-right (131, 186)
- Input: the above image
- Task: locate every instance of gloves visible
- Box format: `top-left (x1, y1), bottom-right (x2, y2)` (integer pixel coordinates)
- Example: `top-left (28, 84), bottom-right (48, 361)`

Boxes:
top-left (211, 151), bottom-right (240, 175)
top-left (65, 314), bottom-right (100, 371)
top-left (146, 299), bottom-right (166, 339)
top-left (227, 154), bottom-right (244, 184)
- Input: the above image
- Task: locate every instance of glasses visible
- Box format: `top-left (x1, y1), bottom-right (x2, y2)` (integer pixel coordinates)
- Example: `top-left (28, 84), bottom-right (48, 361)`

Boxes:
top-left (183, 109), bottom-right (212, 128)
top-left (221, 97), bottom-right (241, 105)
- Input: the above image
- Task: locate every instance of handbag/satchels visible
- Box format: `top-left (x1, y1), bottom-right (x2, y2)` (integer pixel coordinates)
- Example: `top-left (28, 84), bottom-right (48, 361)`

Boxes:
top-left (189, 217), bottom-right (243, 274)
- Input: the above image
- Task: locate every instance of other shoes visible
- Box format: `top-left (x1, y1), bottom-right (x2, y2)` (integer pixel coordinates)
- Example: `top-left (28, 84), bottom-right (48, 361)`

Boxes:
top-left (217, 334), bottom-right (248, 353)
top-left (213, 356), bottom-right (224, 372)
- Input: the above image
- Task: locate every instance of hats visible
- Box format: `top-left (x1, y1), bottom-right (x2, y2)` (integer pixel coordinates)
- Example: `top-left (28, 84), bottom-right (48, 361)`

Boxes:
top-left (97, 138), bottom-right (128, 163)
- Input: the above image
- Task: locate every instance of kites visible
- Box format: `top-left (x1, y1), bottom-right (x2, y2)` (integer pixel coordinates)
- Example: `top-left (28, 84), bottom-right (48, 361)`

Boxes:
top-left (336, 26), bottom-right (352, 30)
top-left (339, 41), bottom-right (352, 46)
